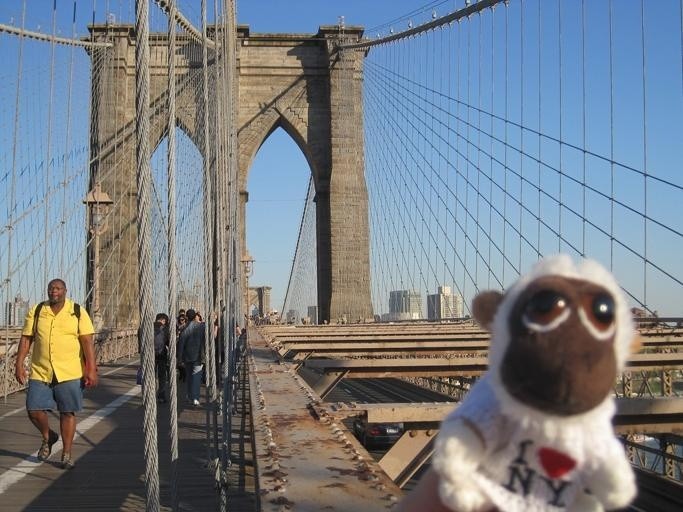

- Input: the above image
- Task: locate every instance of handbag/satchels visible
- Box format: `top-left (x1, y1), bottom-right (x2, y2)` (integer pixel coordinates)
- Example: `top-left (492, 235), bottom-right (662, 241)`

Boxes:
top-left (137, 368), bottom-right (142, 384)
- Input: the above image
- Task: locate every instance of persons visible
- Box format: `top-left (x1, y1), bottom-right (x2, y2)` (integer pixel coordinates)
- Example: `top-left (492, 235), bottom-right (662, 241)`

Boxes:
top-left (135, 306), bottom-right (242, 406)
top-left (15, 277), bottom-right (100, 470)
top-left (391, 465), bottom-right (497, 512)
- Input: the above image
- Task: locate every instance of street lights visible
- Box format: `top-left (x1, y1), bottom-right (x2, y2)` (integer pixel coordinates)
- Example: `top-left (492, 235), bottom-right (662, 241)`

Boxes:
top-left (82, 182), bottom-right (114, 326)
top-left (240, 250), bottom-right (256, 328)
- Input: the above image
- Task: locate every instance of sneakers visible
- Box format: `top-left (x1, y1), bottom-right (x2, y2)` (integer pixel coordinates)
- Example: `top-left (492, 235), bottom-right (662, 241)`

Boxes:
top-left (193, 399), bottom-right (199, 405)
top-left (38, 430), bottom-right (58, 460)
top-left (61, 455), bottom-right (74, 469)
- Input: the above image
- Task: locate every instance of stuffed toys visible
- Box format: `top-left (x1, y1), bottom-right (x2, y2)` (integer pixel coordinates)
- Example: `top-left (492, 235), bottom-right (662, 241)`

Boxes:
top-left (429, 252), bottom-right (644, 512)
